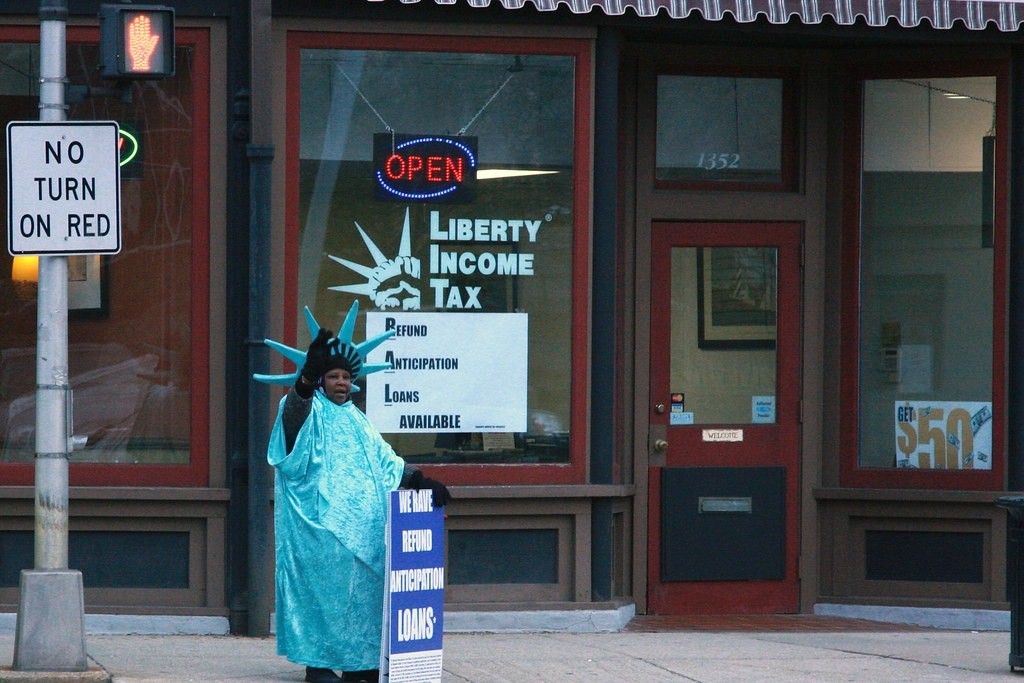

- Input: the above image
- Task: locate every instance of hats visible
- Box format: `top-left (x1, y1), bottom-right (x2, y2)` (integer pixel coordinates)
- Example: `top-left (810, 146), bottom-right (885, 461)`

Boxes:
top-left (253, 300), bottom-right (396, 394)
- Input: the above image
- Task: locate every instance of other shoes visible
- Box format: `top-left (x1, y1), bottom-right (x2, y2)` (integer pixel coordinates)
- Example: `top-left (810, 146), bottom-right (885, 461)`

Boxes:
top-left (306, 670), bottom-right (343, 683)
top-left (342, 668), bottom-right (379, 683)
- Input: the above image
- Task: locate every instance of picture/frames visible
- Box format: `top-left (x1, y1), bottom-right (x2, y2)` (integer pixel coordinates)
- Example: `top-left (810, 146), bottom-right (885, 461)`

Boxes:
top-left (435, 240), bottom-right (519, 313)
top-left (696, 245), bottom-right (777, 350)
top-left (66, 254), bottom-right (110, 320)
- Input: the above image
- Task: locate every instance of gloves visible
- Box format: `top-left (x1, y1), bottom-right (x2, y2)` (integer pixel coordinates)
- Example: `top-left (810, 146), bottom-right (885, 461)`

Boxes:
top-left (408, 470), bottom-right (451, 507)
top-left (301, 327), bottom-right (341, 382)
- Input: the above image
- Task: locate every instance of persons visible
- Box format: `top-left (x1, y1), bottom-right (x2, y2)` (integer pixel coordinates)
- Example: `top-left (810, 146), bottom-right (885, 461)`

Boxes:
top-left (268, 327), bottom-right (452, 682)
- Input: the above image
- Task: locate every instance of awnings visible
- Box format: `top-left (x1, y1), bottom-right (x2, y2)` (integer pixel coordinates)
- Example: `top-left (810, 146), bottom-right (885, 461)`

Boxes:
top-left (376, 0), bottom-right (1024, 32)
